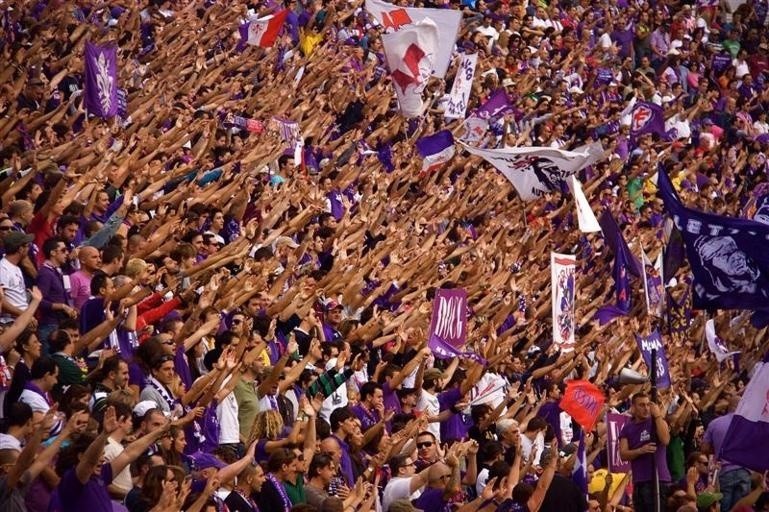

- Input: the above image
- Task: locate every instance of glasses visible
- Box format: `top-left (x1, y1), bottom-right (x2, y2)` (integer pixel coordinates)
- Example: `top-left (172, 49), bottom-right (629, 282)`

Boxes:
top-left (209, 243), bottom-right (218, 247)
top-left (231, 319), bottom-right (244, 325)
top-left (155, 355), bottom-right (174, 366)
top-left (53, 247), bottom-right (68, 253)
top-left (297, 453), bottom-right (305, 461)
top-left (162, 339), bottom-right (176, 345)
top-left (416, 441), bottom-right (435, 449)
top-left (27, 82), bottom-right (45, 87)
top-left (698, 461), bottom-right (709, 466)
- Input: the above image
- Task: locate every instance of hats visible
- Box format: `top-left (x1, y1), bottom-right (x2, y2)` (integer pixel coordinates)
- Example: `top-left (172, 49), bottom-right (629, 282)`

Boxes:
top-left (3, 231), bottom-right (35, 250)
top-left (397, 387), bottom-right (418, 399)
top-left (696, 491), bottom-right (723, 510)
top-left (191, 453), bottom-right (229, 472)
top-left (325, 298), bottom-right (344, 311)
top-left (255, 366), bottom-right (286, 381)
top-left (422, 367), bottom-right (449, 381)
top-left (275, 236), bottom-right (301, 250)
